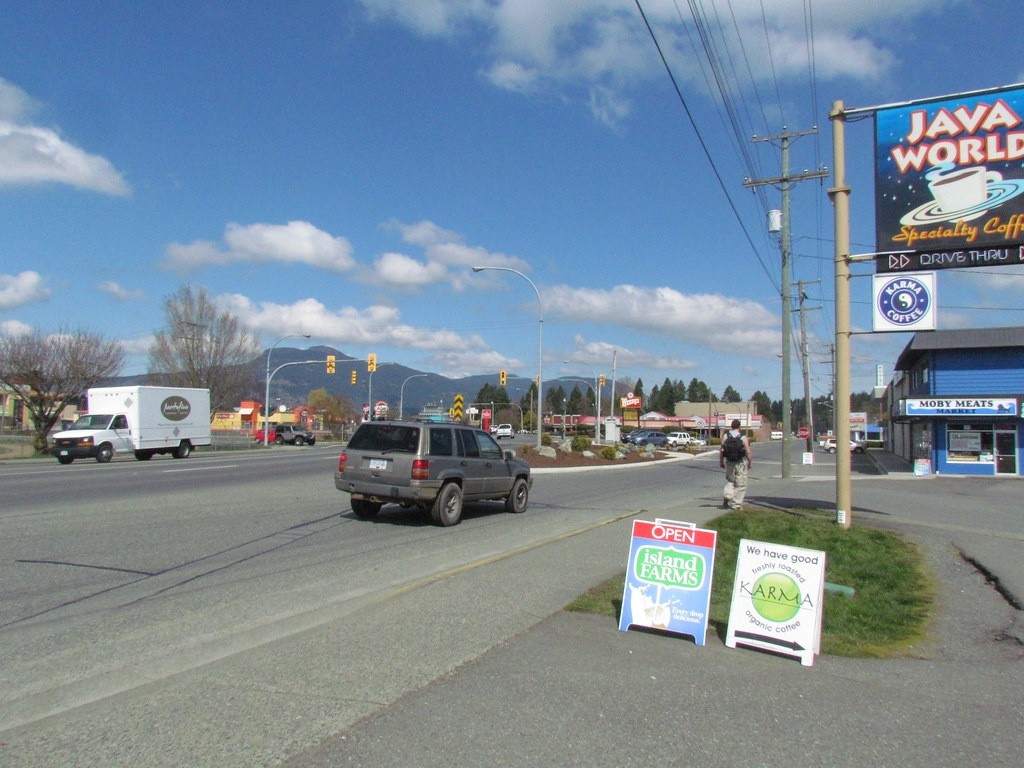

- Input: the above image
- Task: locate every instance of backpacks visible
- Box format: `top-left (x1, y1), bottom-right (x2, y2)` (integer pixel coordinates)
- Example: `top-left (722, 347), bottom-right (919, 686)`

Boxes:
top-left (721, 431), bottom-right (746, 462)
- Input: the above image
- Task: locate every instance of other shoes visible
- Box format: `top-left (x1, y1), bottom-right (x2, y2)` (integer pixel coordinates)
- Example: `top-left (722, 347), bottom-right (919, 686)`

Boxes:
top-left (723, 497), bottom-right (729, 509)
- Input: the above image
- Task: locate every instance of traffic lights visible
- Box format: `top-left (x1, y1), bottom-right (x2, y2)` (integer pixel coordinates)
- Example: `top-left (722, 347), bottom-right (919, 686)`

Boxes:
top-left (351, 370), bottom-right (357, 384)
top-left (500, 371), bottom-right (507, 386)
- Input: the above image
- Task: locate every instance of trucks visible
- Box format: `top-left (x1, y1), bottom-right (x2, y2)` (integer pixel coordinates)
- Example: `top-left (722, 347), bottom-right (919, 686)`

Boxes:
top-left (48, 385), bottom-right (211, 464)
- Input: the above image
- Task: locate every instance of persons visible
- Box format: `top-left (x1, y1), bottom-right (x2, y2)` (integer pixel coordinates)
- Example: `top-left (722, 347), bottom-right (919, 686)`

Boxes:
top-left (719, 419), bottom-right (752, 511)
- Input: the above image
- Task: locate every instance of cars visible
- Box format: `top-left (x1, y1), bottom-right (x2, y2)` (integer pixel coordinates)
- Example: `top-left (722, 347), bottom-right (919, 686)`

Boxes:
top-left (489, 425), bottom-right (498, 434)
top-left (620, 428), bottom-right (707, 448)
top-left (254, 428), bottom-right (276, 444)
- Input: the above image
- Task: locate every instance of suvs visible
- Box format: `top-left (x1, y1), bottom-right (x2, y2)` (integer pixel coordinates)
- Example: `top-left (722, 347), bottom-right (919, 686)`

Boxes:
top-left (824, 437), bottom-right (866, 455)
top-left (334, 414), bottom-right (534, 527)
top-left (274, 424), bottom-right (317, 446)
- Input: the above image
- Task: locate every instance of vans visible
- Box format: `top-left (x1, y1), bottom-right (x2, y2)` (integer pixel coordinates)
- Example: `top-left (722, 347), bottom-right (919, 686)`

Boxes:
top-left (771, 431), bottom-right (783, 440)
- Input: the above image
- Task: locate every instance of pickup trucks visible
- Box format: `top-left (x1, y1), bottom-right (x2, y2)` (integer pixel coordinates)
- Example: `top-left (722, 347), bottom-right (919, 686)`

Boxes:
top-left (496, 423), bottom-right (515, 440)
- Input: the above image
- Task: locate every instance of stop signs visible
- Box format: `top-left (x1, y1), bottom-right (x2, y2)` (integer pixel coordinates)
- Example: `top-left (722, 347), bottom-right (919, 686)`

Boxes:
top-left (798, 427), bottom-right (810, 439)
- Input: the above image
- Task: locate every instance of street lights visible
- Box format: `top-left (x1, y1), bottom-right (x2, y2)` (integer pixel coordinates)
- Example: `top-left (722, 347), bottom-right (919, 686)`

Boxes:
top-left (563, 359), bottom-right (599, 425)
top-left (817, 401), bottom-right (835, 437)
top-left (776, 350), bottom-right (815, 453)
top-left (516, 387), bottom-right (533, 435)
top-left (263, 332), bottom-right (313, 446)
top-left (369, 362), bottom-right (395, 422)
top-left (471, 265), bottom-right (543, 452)
top-left (399, 374), bottom-right (429, 421)
top-left (509, 402), bottom-right (523, 434)
